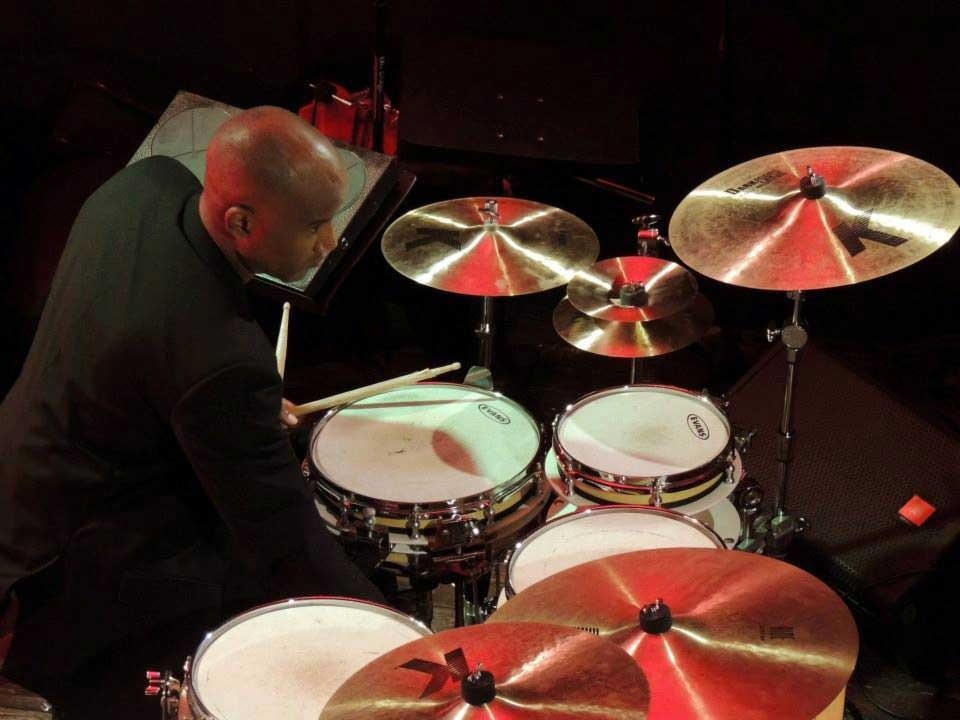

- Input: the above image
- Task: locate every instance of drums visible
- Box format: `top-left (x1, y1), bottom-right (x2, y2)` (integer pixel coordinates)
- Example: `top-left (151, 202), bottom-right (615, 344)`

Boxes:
top-left (540, 486), bottom-right (743, 549)
top-left (177, 589), bottom-right (434, 719)
top-left (549, 379), bottom-right (738, 511)
top-left (295, 376), bottom-right (548, 576)
top-left (493, 504), bottom-right (733, 607)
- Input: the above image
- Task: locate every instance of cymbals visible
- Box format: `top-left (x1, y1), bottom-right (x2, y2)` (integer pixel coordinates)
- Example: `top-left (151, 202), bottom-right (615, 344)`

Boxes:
top-left (550, 284), bottom-right (713, 358)
top-left (317, 619), bottom-right (654, 719)
top-left (378, 193), bottom-right (602, 301)
top-left (566, 254), bottom-right (698, 326)
top-left (475, 543), bottom-right (860, 718)
top-left (663, 144), bottom-right (960, 293)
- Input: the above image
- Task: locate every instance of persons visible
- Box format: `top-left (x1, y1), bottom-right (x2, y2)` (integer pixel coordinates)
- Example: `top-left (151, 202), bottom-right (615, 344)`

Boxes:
top-left (1, 105), bottom-right (384, 719)
